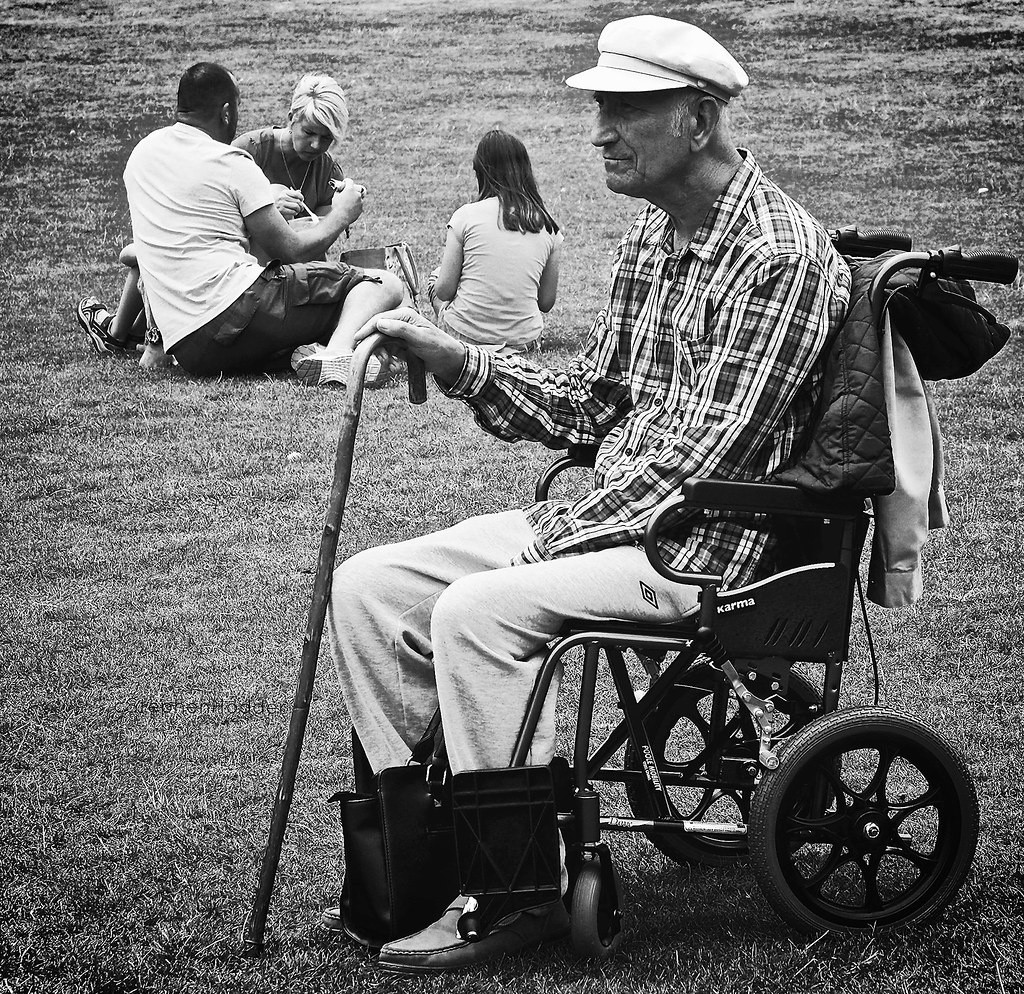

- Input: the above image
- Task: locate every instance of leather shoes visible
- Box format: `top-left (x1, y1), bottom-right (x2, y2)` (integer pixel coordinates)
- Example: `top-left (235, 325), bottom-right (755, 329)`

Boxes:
top-left (319, 905), bottom-right (341, 933)
top-left (375, 894), bottom-right (572, 977)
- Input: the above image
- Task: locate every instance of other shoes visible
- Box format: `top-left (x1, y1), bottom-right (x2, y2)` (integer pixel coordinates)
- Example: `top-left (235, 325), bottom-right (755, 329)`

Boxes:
top-left (290, 342), bottom-right (403, 388)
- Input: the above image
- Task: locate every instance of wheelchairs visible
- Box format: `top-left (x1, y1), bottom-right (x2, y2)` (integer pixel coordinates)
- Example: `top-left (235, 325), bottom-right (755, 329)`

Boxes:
top-left (359, 232), bottom-right (1022, 963)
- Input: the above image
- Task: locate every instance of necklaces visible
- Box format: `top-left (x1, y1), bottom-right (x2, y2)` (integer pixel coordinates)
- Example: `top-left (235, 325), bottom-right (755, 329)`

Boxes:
top-left (279, 129), bottom-right (310, 191)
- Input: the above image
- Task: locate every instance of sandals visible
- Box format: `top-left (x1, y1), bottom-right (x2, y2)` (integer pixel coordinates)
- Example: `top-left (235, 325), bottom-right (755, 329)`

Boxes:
top-left (76, 295), bottom-right (144, 359)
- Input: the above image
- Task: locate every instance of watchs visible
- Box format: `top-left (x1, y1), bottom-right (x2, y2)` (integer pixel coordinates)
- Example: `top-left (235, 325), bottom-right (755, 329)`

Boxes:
top-left (144, 327), bottom-right (162, 345)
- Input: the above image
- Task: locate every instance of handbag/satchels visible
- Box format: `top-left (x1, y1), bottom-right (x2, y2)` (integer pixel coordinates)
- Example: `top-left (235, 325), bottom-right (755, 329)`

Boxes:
top-left (339, 243), bottom-right (422, 315)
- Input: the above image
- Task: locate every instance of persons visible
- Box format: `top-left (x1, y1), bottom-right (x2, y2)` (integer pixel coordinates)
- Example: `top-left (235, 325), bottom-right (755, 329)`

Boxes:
top-left (429, 129), bottom-right (564, 353)
top-left (123, 60), bottom-right (406, 389)
top-left (328, 14), bottom-right (855, 976)
top-left (231, 75), bottom-right (351, 261)
top-left (76, 241), bottom-right (146, 354)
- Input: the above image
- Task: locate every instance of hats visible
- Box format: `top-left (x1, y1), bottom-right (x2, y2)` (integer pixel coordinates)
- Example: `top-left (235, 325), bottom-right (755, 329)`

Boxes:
top-left (564, 13), bottom-right (751, 103)
